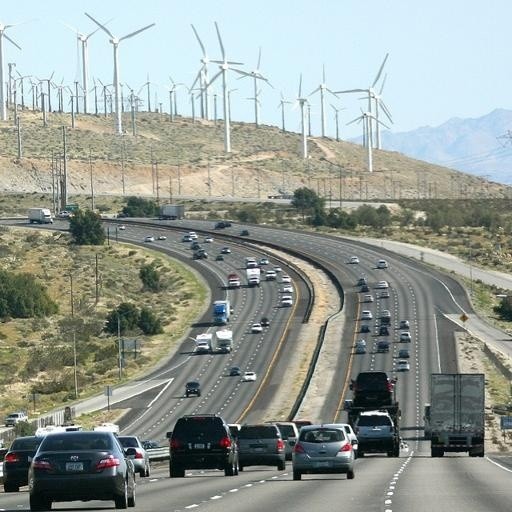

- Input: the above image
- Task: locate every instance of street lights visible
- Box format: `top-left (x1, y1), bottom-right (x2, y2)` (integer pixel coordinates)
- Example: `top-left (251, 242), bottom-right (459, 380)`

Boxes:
top-left (115, 306), bottom-right (144, 382)
top-left (318, 156), bottom-right (346, 210)
top-left (464, 247), bottom-right (475, 293)
top-left (495, 294), bottom-right (512, 300)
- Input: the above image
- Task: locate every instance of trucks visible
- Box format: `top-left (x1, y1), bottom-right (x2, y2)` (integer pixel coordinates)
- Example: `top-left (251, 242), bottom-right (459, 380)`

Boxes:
top-left (158, 203), bottom-right (186, 221)
top-left (427, 372), bottom-right (486, 458)
top-left (26, 206), bottom-right (54, 225)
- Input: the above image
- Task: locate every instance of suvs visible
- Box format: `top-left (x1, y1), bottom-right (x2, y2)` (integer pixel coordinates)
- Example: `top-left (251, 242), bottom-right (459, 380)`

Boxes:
top-left (236, 423), bottom-right (287, 471)
top-left (165, 413), bottom-right (239, 478)
top-left (353, 410), bottom-right (396, 449)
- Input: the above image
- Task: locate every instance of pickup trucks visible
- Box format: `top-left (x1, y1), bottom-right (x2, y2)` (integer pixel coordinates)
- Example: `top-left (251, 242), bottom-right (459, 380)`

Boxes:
top-left (4, 412), bottom-right (28, 428)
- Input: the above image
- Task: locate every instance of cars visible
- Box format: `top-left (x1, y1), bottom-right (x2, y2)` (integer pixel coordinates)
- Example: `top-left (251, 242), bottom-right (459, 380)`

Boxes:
top-left (55, 208), bottom-right (78, 219)
top-left (315, 425), bottom-right (359, 457)
top-left (225, 424), bottom-right (242, 445)
top-left (118, 436), bottom-right (152, 477)
top-left (267, 192), bottom-right (297, 199)
top-left (117, 224), bottom-right (126, 231)
top-left (288, 424), bottom-right (355, 480)
top-left (272, 423), bottom-right (302, 460)
top-left (3, 436), bottom-right (46, 493)
top-left (26, 428), bottom-right (136, 511)
top-left (349, 371), bottom-right (394, 407)
top-left (347, 252), bottom-right (413, 373)
top-left (140, 220), bottom-right (299, 401)
